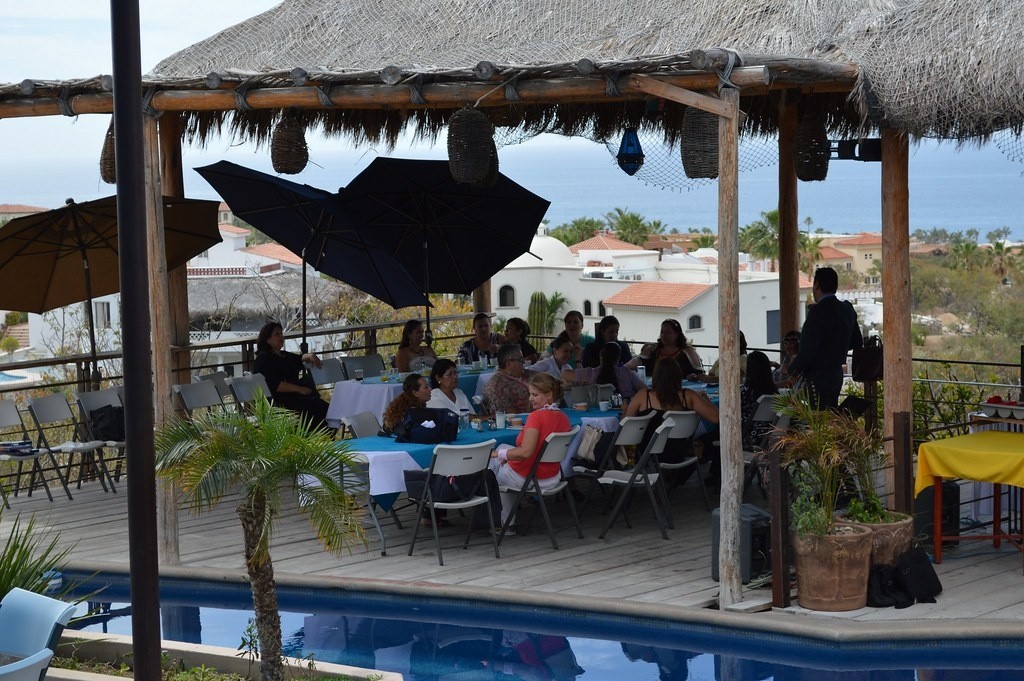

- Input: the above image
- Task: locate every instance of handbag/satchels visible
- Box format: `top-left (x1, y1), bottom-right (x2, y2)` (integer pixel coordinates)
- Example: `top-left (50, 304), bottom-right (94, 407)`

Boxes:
top-left (895, 546), bottom-right (945, 604)
top-left (866, 542), bottom-right (914, 614)
top-left (87, 403), bottom-right (124, 443)
top-left (396, 407), bottom-right (459, 444)
top-left (851, 335), bottom-right (884, 382)
top-left (403, 468), bottom-right (479, 502)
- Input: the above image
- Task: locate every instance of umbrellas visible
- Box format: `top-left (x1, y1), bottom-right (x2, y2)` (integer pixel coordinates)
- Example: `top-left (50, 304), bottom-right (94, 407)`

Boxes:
top-left (192, 156), bottom-right (552, 345)
top-left (0, 194), bottom-right (222, 390)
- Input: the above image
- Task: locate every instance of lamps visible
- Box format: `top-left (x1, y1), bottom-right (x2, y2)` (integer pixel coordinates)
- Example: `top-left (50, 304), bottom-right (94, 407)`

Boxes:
top-left (616, 125), bottom-right (646, 177)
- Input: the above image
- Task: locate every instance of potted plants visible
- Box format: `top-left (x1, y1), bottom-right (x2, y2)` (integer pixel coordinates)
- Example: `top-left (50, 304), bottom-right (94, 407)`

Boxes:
top-left (815, 404), bottom-right (913, 568)
top-left (765, 382), bottom-right (871, 611)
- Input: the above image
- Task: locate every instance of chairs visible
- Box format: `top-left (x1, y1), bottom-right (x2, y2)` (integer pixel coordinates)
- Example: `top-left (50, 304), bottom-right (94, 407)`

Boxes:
top-left (25, 392), bottom-right (108, 502)
top-left (0, 399), bottom-right (52, 509)
top-left (0, 587), bottom-right (78, 681)
top-left (171, 345), bottom-right (790, 567)
top-left (70, 387), bottom-right (124, 493)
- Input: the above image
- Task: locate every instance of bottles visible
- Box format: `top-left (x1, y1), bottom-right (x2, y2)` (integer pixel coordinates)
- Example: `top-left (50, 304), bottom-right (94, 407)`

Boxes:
top-left (630, 366), bottom-right (646, 381)
top-left (612, 393), bottom-right (623, 406)
top-left (488, 411), bottom-right (506, 429)
top-left (458, 353), bottom-right (465, 366)
top-left (415, 357), bottom-right (432, 377)
top-left (460, 409), bottom-right (469, 430)
top-left (471, 418), bottom-right (483, 431)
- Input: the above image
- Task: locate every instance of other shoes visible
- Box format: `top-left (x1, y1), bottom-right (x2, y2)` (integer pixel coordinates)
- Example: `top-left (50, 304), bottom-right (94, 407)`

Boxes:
top-left (489, 526), bottom-right (516, 536)
top-left (424, 519), bottom-right (449, 528)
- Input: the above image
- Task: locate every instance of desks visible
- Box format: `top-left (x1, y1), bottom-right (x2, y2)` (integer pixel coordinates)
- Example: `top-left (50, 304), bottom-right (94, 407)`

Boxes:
top-left (625, 372), bottom-right (720, 474)
top-left (914, 430), bottom-right (1024, 570)
top-left (326, 355), bottom-right (529, 434)
top-left (310, 406), bottom-right (623, 554)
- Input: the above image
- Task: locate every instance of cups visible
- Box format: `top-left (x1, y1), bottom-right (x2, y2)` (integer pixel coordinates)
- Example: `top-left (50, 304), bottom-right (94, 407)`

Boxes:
top-left (511, 418), bottom-right (523, 426)
top-left (399, 373), bottom-right (410, 382)
top-left (599, 402), bottom-right (611, 411)
top-left (505, 413), bottom-right (517, 423)
top-left (472, 361), bottom-right (482, 370)
top-left (390, 368), bottom-right (399, 379)
top-left (491, 358), bottom-right (497, 368)
top-left (573, 403), bottom-right (588, 411)
top-left (380, 370), bottom-right (388, 382)
top-left (355, 370), bottom-right (363, 382)
top-left (479, 355), bottom-right (487, 370)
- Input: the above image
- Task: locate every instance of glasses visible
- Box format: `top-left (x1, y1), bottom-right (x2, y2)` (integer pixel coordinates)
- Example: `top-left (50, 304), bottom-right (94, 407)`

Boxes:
top-left (441, 370), bottom-right (459, 377)
top-left (507, 356), bottom-right (525, 363)
top-left (664, 318), bottom-right (677, 329)
top-left (783, 338), bottom-right (801, 345)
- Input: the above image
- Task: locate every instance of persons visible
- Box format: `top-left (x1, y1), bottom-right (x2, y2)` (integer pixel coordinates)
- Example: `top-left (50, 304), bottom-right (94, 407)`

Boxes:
top-left (251, 321), bottom-right (332, 442)
top-left (455, 314), bottom-right (504, 363)
top-left (490, 634), bottom-right (586, 681)
top-left (583, 317), bottom-right (633, 370)
top-left (384, 374), bottom-right (456, 526)
top-left (772, 331), bottom-right (803, 386)
top-left (689, 329), bottom-right (749, 384)
top-left (499, 318), bottom-right (537, 369)
top-left (620, 642), bottom-right (704, 681)
top-left (428, 359), bottom-right (479, 423)
top-left (787, 267), bottom-right (865, 510)
top-left (488, 373), bottom-right (573, 535)
top-left (483, 344), bottom-right (546, 414)
top-left (564, 343), bottom-right (649, 402)
top-left (706, 349), bottom-right (780, 490)
top-left (643, 319), bottom-right (705, 378)
top-left (522, 311), bottom-right (594, 376)
top-left (623, 357), bottom-right (718, 462)
top-left (395, 318), bottom-right (441, 371)
